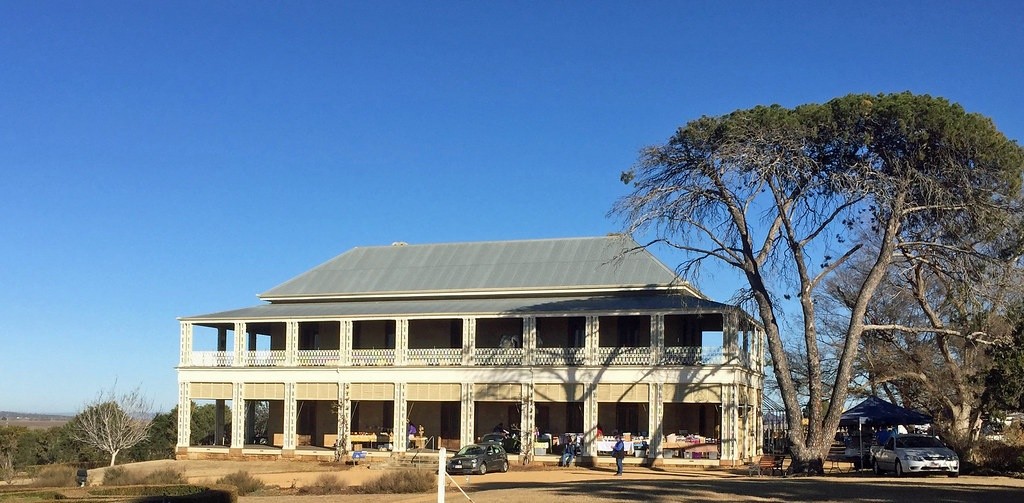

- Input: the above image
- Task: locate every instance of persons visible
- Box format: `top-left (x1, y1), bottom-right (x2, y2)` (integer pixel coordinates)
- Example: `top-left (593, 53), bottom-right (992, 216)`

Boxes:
top-left (598, 422), bottom-right (608, 454)
top-left (861, 422), bottom-right (899, 448)
top-left (611, 435), bottom-right (625, 475)
top-left (493, 423), bottom-right (504, 432)
top-left (406, 418), bottom-right (416, 451)
top-left (561, 434), bottom-right (578, 467)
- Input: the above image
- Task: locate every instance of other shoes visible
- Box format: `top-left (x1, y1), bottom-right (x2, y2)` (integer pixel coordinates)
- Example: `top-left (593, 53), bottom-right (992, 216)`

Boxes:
top-left (614, 474), bottom-right (622, 476)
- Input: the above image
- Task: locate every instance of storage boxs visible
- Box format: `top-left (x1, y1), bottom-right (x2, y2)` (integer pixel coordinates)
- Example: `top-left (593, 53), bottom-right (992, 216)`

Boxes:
top-left (623, 432), bottom-right (717, 460)
top-left (535, 448), bottom-right (546, 455)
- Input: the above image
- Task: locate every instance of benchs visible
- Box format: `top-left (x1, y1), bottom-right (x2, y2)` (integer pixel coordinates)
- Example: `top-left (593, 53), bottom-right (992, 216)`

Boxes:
top-left (748, 455), bottom-right (786, 478)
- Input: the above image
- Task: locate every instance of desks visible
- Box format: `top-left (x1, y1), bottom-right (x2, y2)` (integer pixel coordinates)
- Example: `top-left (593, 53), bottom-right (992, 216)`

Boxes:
top-left (663, 442), bottom-right (685, 459)
top-left (598, 440), bottom-right (635, 456)
top-left (324, 434), bottom-right (428, 449)
top-left (825, 455), bottom-right (861, 474)
top-left (685, 442), bottom-right (717, 460)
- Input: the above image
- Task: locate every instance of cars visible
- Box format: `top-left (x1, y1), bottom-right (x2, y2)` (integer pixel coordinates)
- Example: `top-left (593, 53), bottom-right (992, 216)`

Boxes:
top-left (869, 434), bottom-right (960, 476)
top-left (447, 442), bottom-right (510, 475)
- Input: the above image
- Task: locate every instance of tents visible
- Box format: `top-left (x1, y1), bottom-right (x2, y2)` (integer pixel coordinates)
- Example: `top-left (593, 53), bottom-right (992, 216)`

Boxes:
top-left (837, 396), bottom-right (933, 476)
